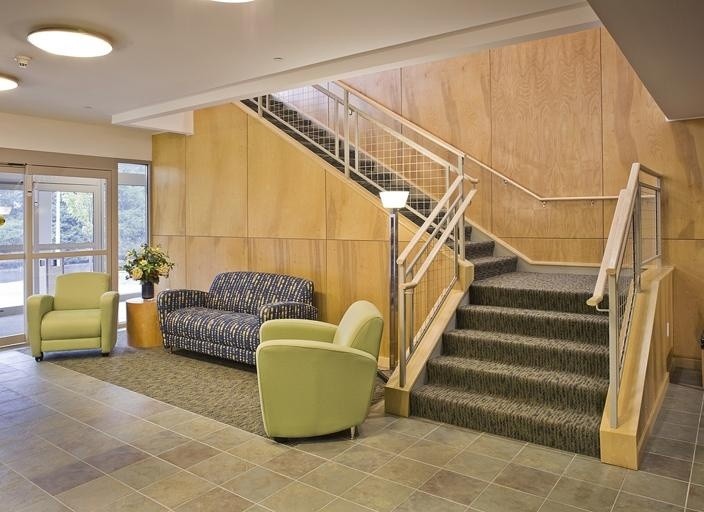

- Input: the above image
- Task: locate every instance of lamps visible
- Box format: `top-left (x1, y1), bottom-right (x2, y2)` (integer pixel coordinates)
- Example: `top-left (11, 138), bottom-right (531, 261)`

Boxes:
top-left (376, 190), bottom-right (409, 383)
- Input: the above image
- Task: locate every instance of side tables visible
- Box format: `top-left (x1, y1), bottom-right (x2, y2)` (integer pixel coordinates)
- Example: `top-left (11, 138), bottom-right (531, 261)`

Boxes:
top-left (126, 297), bottom-right (163, 348)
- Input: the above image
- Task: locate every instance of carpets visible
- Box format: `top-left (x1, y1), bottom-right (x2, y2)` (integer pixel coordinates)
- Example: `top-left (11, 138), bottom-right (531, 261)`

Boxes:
top-left (13, 329), bottom-right (360, 448)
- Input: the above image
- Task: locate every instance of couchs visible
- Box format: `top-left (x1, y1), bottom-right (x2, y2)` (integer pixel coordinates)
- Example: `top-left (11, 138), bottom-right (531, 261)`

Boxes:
top-left (256, 301), bottom-right (384, 443)
top-left (157, 272), bottom-right (319, 366)
top-left (25, 272), bottom-right (119, 362)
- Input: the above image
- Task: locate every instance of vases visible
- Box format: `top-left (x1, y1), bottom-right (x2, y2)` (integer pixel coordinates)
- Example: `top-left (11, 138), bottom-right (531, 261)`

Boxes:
top-left (142, 281), bottom-right (155, 300)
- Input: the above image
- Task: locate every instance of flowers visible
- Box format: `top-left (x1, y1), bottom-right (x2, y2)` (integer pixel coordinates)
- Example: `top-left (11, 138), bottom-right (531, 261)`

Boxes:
top-left (122, 242), bottom-right (175, 285)
